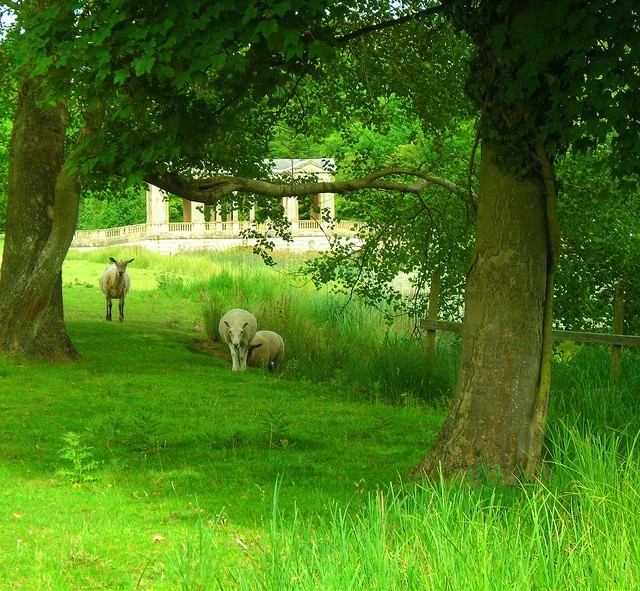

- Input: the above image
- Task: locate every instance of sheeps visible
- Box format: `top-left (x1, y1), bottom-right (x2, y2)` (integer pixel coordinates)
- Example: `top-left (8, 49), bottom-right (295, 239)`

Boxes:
top-left (218, 308), bottom-right (258, 371)
top-left (246, 330), bottom-right (286, 371)
top-left (98, 257), bottom-right (135, 323)
